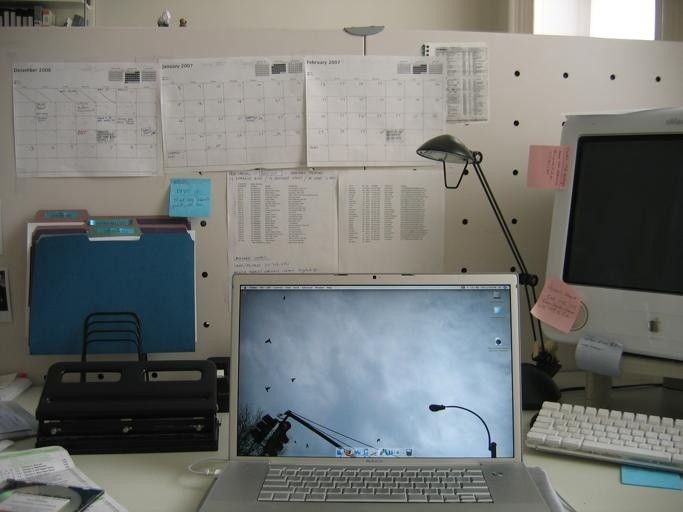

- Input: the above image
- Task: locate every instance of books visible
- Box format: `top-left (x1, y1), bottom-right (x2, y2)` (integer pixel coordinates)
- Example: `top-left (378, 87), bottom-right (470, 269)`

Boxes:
top-left (0, 5), bottom-right (88, 27)
top-left (0, 476), bottom-right (105, 511)
top-left (1, 371), bottom-right (33, 402)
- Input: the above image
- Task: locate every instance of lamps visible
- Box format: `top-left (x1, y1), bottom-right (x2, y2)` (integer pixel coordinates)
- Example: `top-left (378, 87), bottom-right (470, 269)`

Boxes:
top-left (416, 134), bottom-right (563, 410)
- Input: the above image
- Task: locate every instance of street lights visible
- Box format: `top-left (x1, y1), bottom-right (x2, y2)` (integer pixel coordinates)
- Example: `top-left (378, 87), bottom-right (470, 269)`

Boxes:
top-left (428, 404), bottom-right (497, 457)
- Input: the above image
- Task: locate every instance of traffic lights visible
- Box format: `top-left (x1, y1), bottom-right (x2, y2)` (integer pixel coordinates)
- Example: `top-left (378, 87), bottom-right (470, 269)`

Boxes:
top-left (264, 422), bottom-right (292, 456)
top-left (251, 414), bottom-right (274, 442)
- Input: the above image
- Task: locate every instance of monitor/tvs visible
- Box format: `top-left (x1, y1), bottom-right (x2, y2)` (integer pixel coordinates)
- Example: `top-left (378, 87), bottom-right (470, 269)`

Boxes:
top-left (540, 109), bottom-right (683, 361)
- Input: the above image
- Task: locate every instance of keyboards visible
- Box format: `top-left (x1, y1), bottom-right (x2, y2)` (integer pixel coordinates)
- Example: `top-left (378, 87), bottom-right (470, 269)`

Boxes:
top-left (525, 400), bottom-right (683, 472)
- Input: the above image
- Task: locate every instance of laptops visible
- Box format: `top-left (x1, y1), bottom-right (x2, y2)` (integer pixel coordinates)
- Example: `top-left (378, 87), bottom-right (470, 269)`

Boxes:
top-left (197, 272), bottom-right (551, 512)
